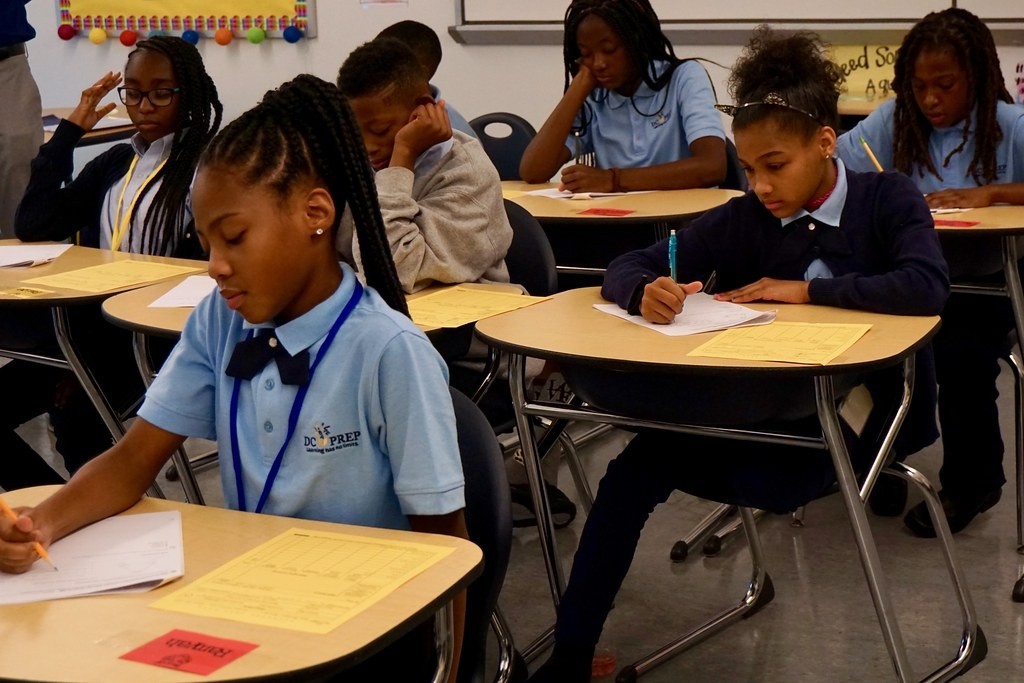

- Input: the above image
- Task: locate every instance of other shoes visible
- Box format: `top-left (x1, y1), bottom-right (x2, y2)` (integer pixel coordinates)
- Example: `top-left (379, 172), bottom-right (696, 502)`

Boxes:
top-left (869, 458), bottom-right (908, 517)
top-left (526, 641), bottom-right (597, 683)
top-left (903, 485), bottom-right (1003, 539)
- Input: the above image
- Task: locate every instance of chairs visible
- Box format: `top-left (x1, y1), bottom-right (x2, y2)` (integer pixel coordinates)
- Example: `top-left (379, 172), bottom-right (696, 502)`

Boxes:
top-left (1, 111), bottom-right (1023, 682)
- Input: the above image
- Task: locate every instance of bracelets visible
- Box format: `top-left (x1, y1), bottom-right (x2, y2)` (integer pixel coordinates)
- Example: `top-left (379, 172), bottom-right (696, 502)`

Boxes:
top-left (609, 167), bottom-right (621, 191)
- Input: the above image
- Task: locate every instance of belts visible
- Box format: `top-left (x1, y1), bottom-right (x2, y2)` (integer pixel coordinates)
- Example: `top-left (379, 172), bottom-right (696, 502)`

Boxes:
top-left (0, 42), bottom-right (26, 63)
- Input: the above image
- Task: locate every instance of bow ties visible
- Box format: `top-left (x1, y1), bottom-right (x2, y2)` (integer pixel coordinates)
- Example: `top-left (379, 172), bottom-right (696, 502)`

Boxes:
top-left (224, 330), bottom-right (311, 386)
top-left (772, 215), bottom-right (849, 259)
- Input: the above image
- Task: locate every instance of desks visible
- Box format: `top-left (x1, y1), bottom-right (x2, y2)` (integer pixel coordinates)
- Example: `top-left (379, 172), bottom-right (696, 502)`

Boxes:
top-left (0, 485), bottom-right (485, 683)
top-left (101, 270), bottom-right (555, 682)
top-left (42, 103), bottom-right (137, 150)
top-left (923, 191), bottom-right (1024, 604)
top-left (0, 237), bottom-right (214, 501)
top-left (474, 285), bottom-right (988, 683)
top-left (502, 179), bottom-right (746, 244)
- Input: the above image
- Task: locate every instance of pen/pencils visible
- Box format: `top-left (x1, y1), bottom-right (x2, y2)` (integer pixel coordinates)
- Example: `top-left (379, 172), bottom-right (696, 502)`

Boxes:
top-left (858, 136), bottom-right (883, 172)
top-left (669, 229), bottom-right (677, 280)
top-left (575, 130), bottom-right (580, 164)
top-left (0, 496), bottom-right (58, 571)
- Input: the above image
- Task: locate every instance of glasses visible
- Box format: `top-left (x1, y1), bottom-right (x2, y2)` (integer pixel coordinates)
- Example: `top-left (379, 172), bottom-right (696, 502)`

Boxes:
top-left (116, 84), bottom-right (180, 107)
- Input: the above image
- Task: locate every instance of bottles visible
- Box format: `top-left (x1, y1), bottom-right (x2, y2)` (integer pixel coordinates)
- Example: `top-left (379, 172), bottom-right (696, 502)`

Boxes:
top-left (592, 614), bottom-right (617, 675)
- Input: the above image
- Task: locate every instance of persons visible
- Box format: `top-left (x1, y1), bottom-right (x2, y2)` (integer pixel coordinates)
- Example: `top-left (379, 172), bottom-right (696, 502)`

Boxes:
top-left (0, 0), bottom-right (44, 241)
top-left (519, 0), bottom-right (727, 394)
top-left (521, 28), bottom-right (949, 683)
top-left (0, 36), bottom-right (223, 488)
top-left (836, 8), bottom-right (1024, 538)
top-left (337, 38), bottom-right (576, 527)
top-left (377, 20), bottom-right (479, 137)
top-left (0, 73), bottom-right (466, 683)
